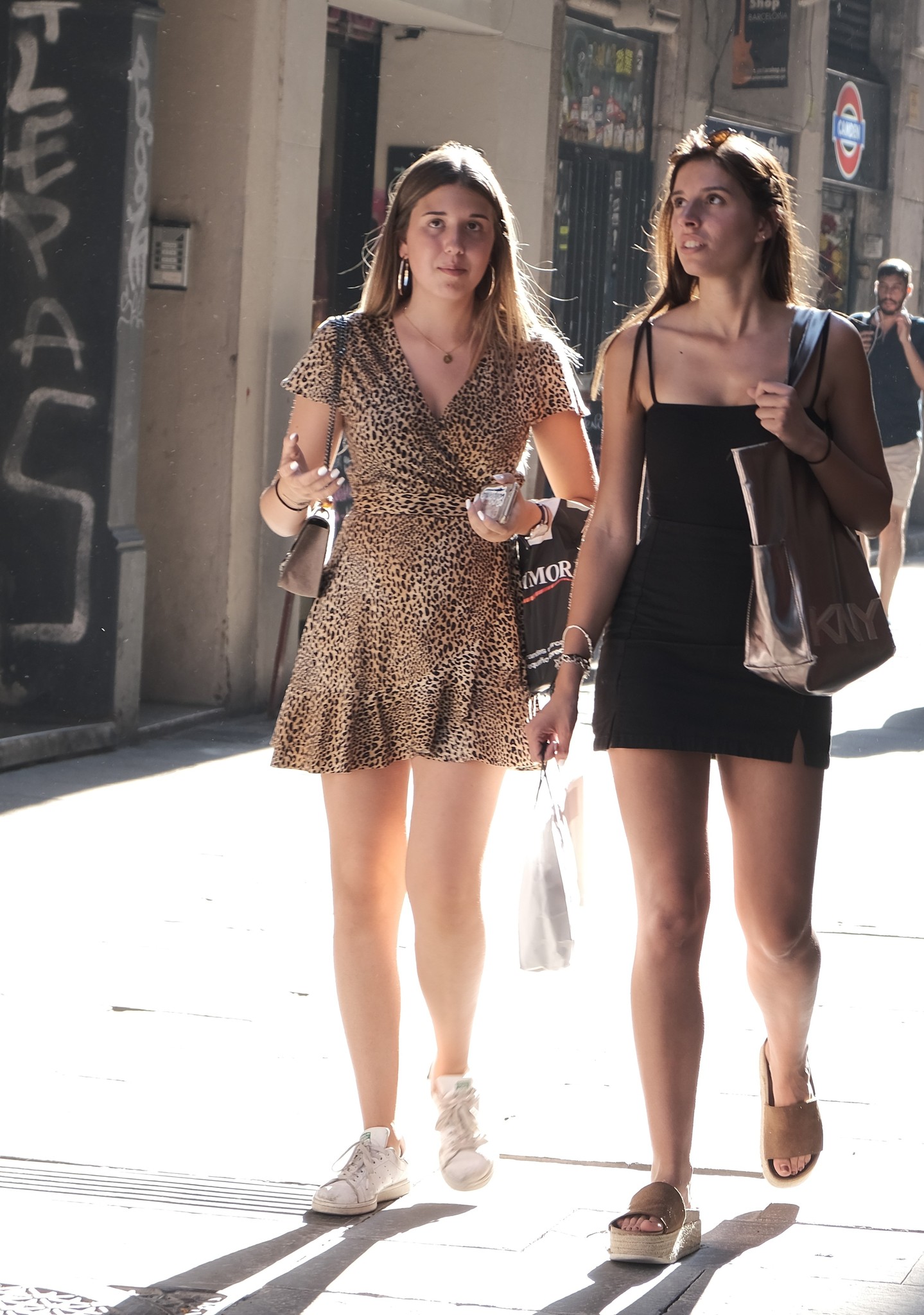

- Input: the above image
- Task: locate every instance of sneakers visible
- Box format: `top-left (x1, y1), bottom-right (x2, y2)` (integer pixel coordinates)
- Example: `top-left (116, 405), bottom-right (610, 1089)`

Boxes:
top-left (311, 1128), bottom-right (411, 1215)
top-left (430, 1066), bottom-right (497, 1189)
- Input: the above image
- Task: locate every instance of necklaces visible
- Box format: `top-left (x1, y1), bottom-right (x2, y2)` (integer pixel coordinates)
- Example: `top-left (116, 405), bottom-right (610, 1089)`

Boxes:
top-left (400, 302), bottom-right (480, 364)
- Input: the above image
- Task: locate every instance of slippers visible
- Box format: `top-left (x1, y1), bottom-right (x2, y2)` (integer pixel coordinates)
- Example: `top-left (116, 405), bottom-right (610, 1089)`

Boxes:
top-left (611, 1180), bottom-right (701, 1264)
top-left (762, 1040), bottom-right (825, 1180)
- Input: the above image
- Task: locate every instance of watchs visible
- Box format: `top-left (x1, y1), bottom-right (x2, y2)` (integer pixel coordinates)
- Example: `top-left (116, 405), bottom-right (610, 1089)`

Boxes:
top-left (522, 502), bottom-right (548, 542)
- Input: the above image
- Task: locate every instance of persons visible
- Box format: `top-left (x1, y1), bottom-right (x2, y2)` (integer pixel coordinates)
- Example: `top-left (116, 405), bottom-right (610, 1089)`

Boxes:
top-left (520, 121), bottom-right (896, 1268)
top-left (257, 138), bottom-right (602, 1220)
top-left (847, 258), bottom-right (924, 626)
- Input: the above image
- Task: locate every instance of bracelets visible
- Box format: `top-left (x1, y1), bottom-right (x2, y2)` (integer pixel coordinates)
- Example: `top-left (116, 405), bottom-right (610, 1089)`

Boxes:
top-left (554, 624), bottom-right (594, 681)
top-left (807, 434), bottom-right (832, 465)
top-left (275, 479), bottom-right (312, 514)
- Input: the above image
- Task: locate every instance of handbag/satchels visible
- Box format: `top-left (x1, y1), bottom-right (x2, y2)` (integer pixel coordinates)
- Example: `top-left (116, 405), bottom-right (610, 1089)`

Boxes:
top-left (515, 497), bottom-right (594, 696)
top-left (730, 307), bottom-right (897, 697)
top-left (278, 315), bottom-right (348, 599)
top-left (518, 748), bottom-right (583, 972)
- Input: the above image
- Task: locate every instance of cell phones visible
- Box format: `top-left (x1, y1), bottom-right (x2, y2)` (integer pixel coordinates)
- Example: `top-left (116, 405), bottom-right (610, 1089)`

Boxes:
top-left (478, 482), bottom-right (520, 525)
